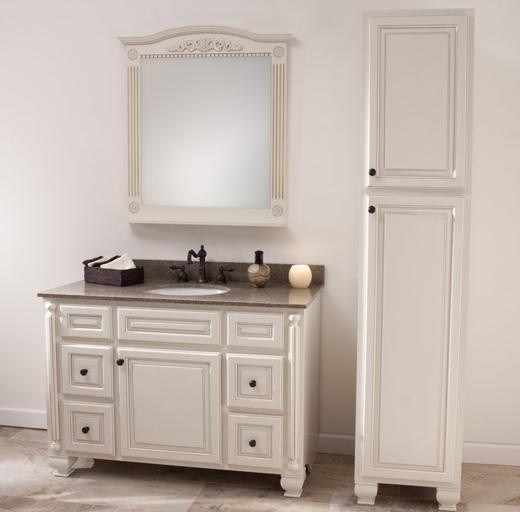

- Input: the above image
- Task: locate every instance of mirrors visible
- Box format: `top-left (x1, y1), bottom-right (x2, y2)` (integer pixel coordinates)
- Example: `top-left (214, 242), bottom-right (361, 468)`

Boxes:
top-left (119, 25), bottom-right (293, 227)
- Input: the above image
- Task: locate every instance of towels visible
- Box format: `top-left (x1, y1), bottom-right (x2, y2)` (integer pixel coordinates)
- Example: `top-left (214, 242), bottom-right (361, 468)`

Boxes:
top-left (82, 255), bottom-right (136, 270)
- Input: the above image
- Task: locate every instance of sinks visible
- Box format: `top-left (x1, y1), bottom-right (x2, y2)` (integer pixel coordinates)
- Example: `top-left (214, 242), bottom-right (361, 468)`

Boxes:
top-left (143, 283), bottom-right (230, 295)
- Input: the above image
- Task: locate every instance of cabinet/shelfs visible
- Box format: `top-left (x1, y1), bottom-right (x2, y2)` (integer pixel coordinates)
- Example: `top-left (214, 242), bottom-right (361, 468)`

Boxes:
top-left (42, 294), bottom-right (321, 498)
top-left (353, 14), bottom-right (472, 511)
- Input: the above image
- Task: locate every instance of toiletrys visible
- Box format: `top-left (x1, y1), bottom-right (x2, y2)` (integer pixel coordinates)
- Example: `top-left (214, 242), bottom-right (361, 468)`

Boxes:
top-left (246, 250), bottom-right (273, 289)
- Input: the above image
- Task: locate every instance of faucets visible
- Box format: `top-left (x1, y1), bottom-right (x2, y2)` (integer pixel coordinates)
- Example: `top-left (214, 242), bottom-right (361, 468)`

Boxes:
top-left (186, 245), bottom-right (208, 282)
